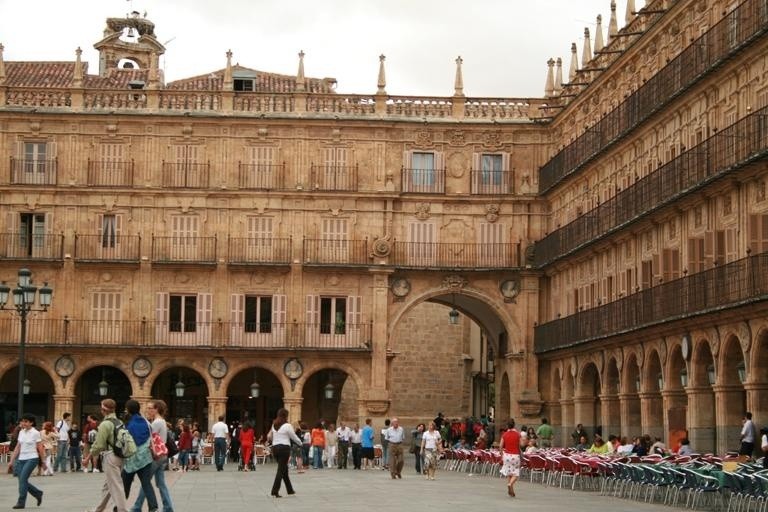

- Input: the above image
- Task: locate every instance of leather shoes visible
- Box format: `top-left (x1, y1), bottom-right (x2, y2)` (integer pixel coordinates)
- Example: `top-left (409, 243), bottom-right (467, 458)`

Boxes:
top-left (13, 503), bottom-right (24, 508)
top-left (36, 491), bottom-right (43, 505)
top-left (391, 472), bottom-right (396, 478)
top-left (398, 473), bottom-right (401, 478)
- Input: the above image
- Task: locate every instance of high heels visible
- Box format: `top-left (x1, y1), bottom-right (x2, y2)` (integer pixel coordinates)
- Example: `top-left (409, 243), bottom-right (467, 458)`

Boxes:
top-left (287, 490), bottom-right (295, 494)
top-left (507, 483), bottom-right (512, 494)
top-left (512, 491), bottom-right (515, 496)
top-left (271, 491), bottom-right (282, 497)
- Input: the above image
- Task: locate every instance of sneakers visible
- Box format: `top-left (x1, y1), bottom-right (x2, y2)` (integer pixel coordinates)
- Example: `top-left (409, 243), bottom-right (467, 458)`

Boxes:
top-left (288, 464), bottom-right (381, 473)
top-left (33, 467), bottom-right (100, 476)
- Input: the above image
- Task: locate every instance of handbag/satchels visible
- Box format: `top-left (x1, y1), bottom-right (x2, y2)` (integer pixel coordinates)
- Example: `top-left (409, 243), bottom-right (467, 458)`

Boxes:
top-left (149, 432), bottom-right (169, 460)
top-left (266, 430), bottom-right (273, 445)
top-left (123, 439), bottom-right (154, 473)
top-left (167, 435), bottom-right (179, 457)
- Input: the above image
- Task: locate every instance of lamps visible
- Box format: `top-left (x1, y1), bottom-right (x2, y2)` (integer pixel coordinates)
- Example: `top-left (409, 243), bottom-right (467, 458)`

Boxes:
top-left (636, 361), bottom-right (745, 391)
top-left (449, 293), bottom-right (459, 323)
top-left (250, 367), bottom-right (260, 398)
top-left (175, 367), bottom-right (185, 397)
top-left (23, 366), bottom-right (32, 394)
top-left (98, 366), bottom-right (109, 396)
top-left (324, 370), bottom-right (335, 399)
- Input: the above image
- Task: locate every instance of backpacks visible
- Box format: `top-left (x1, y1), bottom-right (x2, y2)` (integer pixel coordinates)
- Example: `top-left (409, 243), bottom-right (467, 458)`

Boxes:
top-left (104, 418), bottom-right (137, 457)
top-left (88, 423), bottom-right (97, 443)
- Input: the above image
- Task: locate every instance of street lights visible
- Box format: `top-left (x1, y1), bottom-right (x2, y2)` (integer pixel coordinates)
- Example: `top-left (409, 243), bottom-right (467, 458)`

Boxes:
top-left (0, 268), bottom-right (53, 420)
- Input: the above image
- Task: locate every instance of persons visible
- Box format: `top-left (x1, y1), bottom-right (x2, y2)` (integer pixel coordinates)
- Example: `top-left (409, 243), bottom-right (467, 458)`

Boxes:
top-left (7, 414), bottom-right (47, 509)
top-left (266, 408), bottom-right (303, 499)
top-left (82, 399), bottom-right (129, 512)
top-left (571, 416), bottom-right (694, 457)
top-left (499, 418), bottom-right (534, 498)
top-left (7, 413), bottom-right (496, 477)
top-left (128, 400), bottom-right (175, 512)
top-left (738, 413), bottom-right (755, 458)
top-left (761, 427), bottom-right (768, 470)
top-left (498, 418), bottom-right (556, 453)
top-left (112, 400), bottom-right (160, 512)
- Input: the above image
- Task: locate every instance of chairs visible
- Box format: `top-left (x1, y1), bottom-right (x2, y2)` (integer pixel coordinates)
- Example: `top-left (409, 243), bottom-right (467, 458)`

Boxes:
top-left (201, 446), bottom-right (214, 465)
top-left (255, 446), bottom-right (268, 465)
top-left (0, 441), bottom-right (13, 463)
top-left (373, 446), bottom-right (382, 467)
